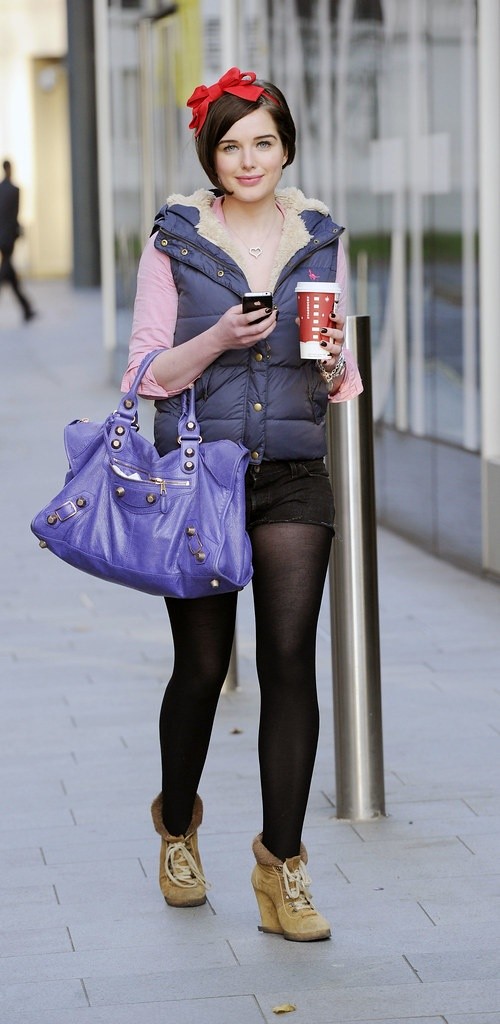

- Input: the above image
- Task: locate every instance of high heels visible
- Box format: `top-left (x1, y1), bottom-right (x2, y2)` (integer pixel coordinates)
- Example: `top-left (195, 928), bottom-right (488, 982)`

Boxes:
top-left (251, 831), bottom-right (331, 941)
top-left (151, 790), bottom-right (213, 906)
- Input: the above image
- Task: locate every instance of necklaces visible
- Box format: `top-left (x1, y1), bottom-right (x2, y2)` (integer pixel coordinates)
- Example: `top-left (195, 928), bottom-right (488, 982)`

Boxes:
top-left (223, 200), bottom-right (278, 259)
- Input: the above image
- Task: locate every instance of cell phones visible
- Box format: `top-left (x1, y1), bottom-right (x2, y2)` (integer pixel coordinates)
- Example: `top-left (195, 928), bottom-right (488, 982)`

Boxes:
top-left (242, 291), bottom-right (273, 325)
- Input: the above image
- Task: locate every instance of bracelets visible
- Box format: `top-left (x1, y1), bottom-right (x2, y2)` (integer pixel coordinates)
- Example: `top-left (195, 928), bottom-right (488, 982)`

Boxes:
top-left (315, 351), bottom-right (347, 393)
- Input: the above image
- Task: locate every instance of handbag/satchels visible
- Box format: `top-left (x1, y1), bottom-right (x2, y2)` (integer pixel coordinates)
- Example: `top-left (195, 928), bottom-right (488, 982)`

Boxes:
top-left (31, 348), bottom-right (254, 598)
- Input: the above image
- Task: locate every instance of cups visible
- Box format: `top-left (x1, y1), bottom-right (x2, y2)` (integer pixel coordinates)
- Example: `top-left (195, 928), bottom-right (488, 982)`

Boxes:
top-left (295, 281), bottom-right (342, 359)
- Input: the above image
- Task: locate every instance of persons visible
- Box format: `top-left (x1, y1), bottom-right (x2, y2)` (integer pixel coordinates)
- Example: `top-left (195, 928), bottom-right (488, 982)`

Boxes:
top-left (120, 72), bottom-right (364, 944)
top-left (0, 160), bottom-right (40, 325)
